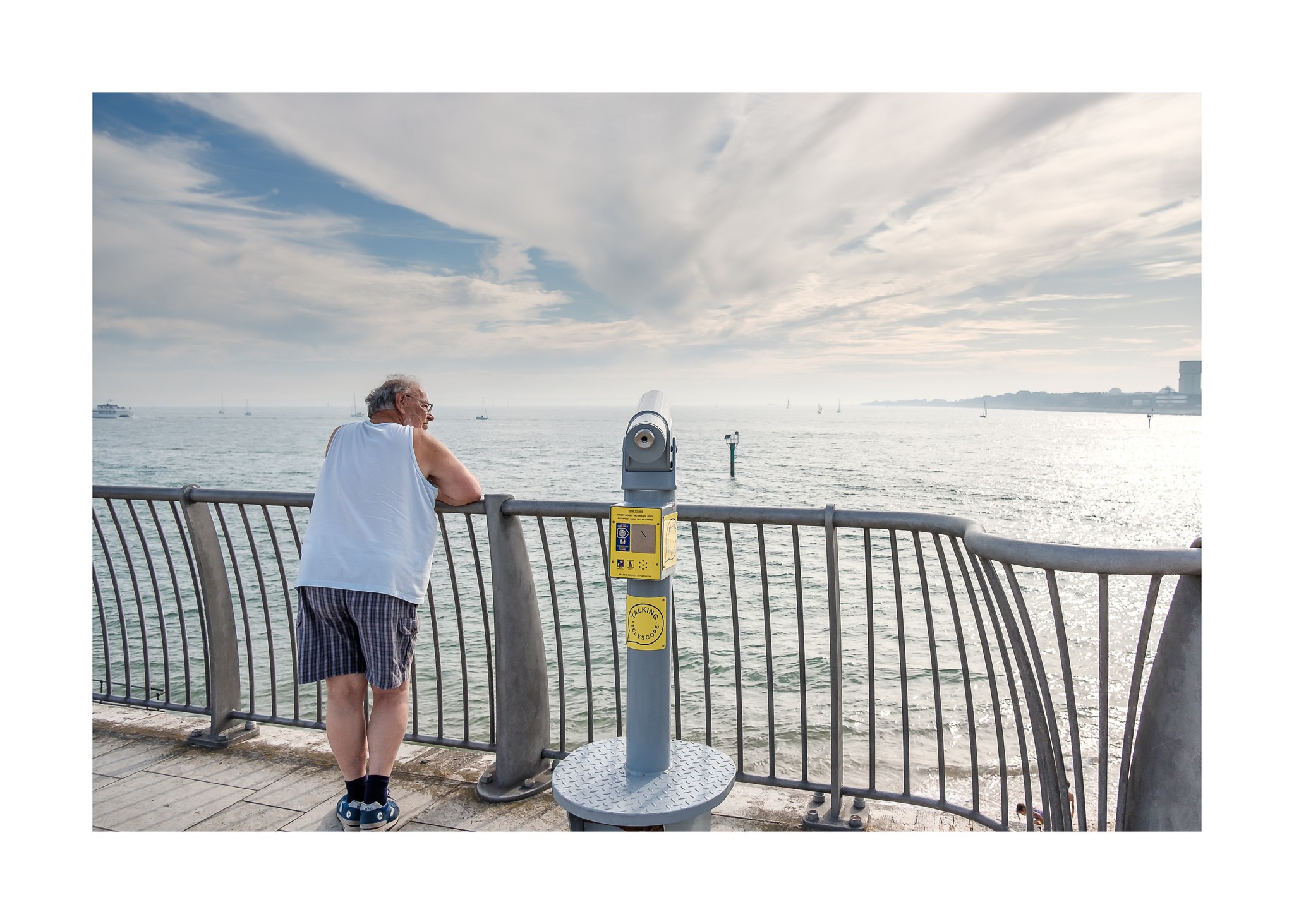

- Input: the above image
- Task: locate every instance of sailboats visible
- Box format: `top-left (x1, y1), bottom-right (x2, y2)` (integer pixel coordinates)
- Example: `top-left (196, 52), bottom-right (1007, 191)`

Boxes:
top-left (350, 391), bottom-right (365, 417)
top-left (786, 398), bottom-right (790, 409)
top-left (980, 401), bottom-right (987, 417)
top-left (837, 399), bottom-right (841, 413)
top-left (476, 397), bottom-right (488, 420)
top-left (245, 399), bottom-right (252, 416)
top-left (218, 393), bottom-right (224, 414)
top-left (817, 404), bottom-right (822, 413)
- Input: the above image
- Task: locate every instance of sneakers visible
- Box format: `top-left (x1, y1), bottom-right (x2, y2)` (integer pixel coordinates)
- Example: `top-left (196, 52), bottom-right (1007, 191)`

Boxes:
top-left (335, 793), bottom-right (363, 832)
top-left (359, 789), bottom-right (400, 833)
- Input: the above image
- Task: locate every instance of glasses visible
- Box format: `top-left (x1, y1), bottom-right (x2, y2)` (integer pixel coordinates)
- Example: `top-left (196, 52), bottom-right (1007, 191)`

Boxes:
top-left (405, 394), bottom-right (433, 416)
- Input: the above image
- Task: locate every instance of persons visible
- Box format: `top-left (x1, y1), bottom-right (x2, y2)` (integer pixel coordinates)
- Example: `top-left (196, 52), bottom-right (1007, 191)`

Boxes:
top-left (295, 374), bottom-right (482, 830)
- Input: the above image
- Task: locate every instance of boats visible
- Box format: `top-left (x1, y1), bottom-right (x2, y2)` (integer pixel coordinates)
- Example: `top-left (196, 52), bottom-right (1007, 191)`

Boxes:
top-left (92, 398), bottom-right (135, 419)
